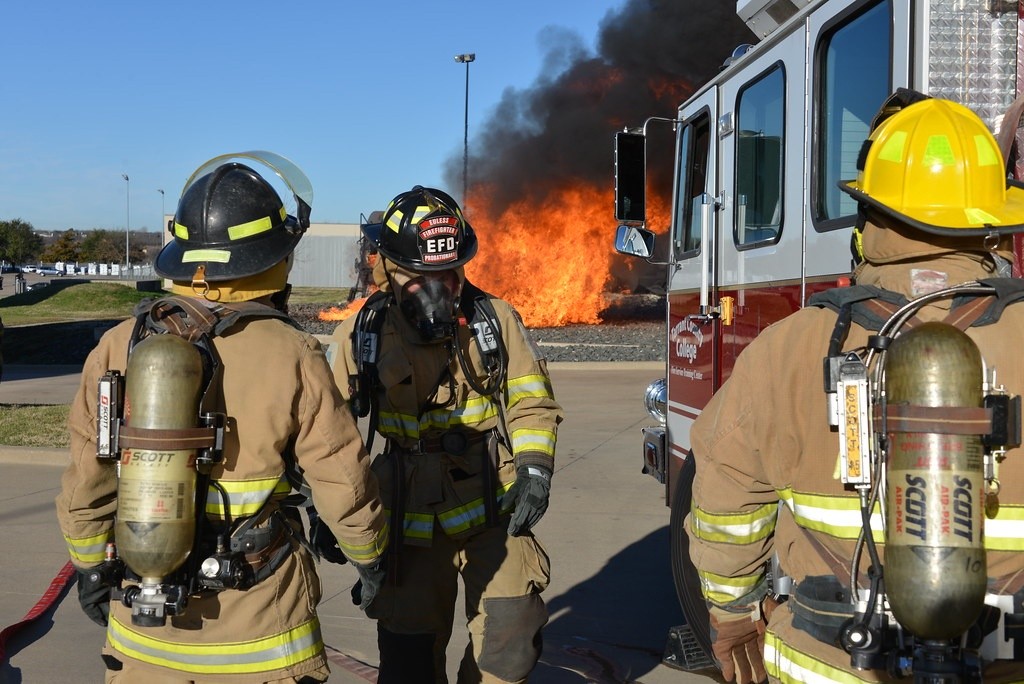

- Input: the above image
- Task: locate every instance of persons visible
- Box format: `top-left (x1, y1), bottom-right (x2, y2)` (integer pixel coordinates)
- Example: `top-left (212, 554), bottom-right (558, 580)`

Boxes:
top-left (310, 186), bottom-right (565, 684)
top-left (684, 98), bottom-right (1024, 684)
top-left (55, 151), bottom-right (390, 684)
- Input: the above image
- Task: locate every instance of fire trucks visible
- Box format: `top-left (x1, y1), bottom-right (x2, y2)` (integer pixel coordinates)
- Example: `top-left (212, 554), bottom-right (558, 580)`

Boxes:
top-left (611, 1), bottom-right (1024, 684)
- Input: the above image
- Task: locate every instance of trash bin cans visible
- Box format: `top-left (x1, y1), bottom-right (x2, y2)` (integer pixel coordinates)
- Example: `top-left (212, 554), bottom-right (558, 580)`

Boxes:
top-left (14, 275), bottom-right (24, 295)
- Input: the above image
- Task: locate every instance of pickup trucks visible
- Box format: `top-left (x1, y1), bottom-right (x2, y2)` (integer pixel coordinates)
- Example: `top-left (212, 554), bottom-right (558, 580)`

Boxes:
top-left (22, 264), bottom-right (37, 273)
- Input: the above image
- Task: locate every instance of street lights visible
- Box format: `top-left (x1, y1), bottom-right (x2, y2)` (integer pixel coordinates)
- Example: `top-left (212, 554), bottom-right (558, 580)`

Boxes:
top-left (154, 189), bottom-right (165, 250)
top-left (122, 172), bottom-right (133, 271)
top-left (456, 50), bottom-right (481, 217)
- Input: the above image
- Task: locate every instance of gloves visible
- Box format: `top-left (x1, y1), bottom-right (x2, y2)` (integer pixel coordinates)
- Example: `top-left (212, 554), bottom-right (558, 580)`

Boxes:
top-left (348, 557), bottom-right (391, 610)
top-left (77, 562), bottom-right (112, 626)
top-left (706, 601), bottom-right (766, 684)
top-left (306, 505), bottom-right (348, 565)
top-left (501, 451), bottom-right (554, 537)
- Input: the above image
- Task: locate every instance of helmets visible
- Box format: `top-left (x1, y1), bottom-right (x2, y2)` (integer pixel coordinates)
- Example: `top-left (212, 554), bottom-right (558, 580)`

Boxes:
top-left (154, 163), bottom-right (306, 281)
top-left (837, 98), bottom-right (1024, 237)
top-left (360, 185), bottom-right (478, 271)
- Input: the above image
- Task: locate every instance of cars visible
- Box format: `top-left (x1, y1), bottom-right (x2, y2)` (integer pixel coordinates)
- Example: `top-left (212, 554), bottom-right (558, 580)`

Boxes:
top-left (36, 267), bottom-right (65, 277)
top-left (64, 265), bottom-right (80, 275)
